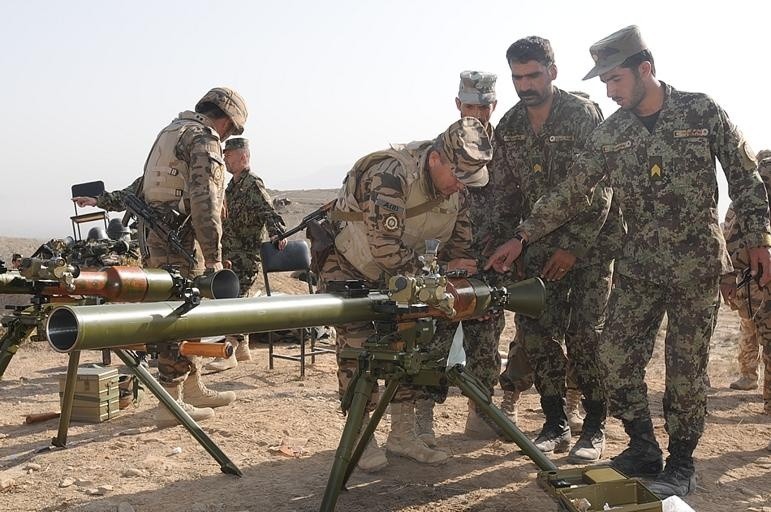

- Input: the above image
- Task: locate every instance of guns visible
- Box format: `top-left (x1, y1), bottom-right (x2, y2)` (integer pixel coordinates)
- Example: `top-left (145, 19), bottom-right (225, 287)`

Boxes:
top-left (271, 199), bottom-right (338, 245)
top-left (121, 192), bottom-right (198, 270)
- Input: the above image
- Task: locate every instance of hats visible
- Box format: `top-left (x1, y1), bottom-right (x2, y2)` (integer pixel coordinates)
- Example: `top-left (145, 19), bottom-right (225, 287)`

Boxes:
top-left (436, 115), bottom-right (494, 188)
top-left (223, 138), bottom-right (251, 153)
top-left (582, 25), bottom-right (648, 81)
top-left (459, 70), bottom-right (498, 106)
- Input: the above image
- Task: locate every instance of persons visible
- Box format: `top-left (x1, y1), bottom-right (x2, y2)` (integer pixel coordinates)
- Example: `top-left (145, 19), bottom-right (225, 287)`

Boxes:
top-left (412, 69), bottom-right (506, 448)
top-left (315, 115), bottom-right (495, 474)
top-left (12, 253), bottom-right (24, 269)
top-left (492, 35), bottom-right (627, 466)
top-left (747, 156), bottom-right (771, 412)
top-left (722, 149), bottom-right (771, 391)
top-left (482, 25), bottom-right (771, 499)
top-left (500, 90), bottom-right (602, 435)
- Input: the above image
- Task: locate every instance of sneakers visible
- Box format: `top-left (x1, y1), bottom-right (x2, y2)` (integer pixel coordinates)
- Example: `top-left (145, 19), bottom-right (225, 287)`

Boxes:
top-left (729, 373), bottom-right (758, 390)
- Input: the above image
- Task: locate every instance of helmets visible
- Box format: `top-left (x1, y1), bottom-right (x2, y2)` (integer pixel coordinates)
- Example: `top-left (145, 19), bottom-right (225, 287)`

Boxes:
top-left (88, 218), bottom-right (132, 241)
top-left (195, 87), bottom-right (248, 135)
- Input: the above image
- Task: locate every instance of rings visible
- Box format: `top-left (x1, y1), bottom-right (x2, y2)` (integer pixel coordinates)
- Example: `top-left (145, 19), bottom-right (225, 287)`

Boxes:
top-left (559, 269), bottom-right (565, 272)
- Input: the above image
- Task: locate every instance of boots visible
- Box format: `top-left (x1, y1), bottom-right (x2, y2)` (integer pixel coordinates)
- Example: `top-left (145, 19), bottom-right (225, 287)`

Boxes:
top-left (464, 390), bottom-right (522, 442)
top-left (235, 335), bottom-right (251, 361)
top-left (155, 381), bottom-right (215, 429)
top-left (205, 337), bottom-right (240, 370)
top-left (520, 388), bottom-right (607, 464)
top-left (608, 419), bottom-right (663, 478)
top-left (183, 370), bottom-right (236, 408)
top-left (351, 412), bottom-right (390, 474)
top-left (386, 398), bottom-right (449, 467)
top-left (639, 438), bottom-right (698, 500)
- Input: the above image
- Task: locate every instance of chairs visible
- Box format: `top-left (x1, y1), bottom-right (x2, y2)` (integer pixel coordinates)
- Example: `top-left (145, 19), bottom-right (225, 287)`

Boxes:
top-left (68, 178), bottom-right (109, 244)
top-left (260, 240), bottom-right (339, 377)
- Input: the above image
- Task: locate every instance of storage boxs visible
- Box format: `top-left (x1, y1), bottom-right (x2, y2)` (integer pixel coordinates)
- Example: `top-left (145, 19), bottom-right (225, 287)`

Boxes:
top-left (534, 463), bottom-right (665, 512)
top-left (57, 363), bottom-right (118, 423)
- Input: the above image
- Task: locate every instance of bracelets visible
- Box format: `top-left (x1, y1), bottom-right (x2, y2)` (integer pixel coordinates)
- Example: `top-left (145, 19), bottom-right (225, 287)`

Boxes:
top-left (513, 233), bottom-right (526, 252)
